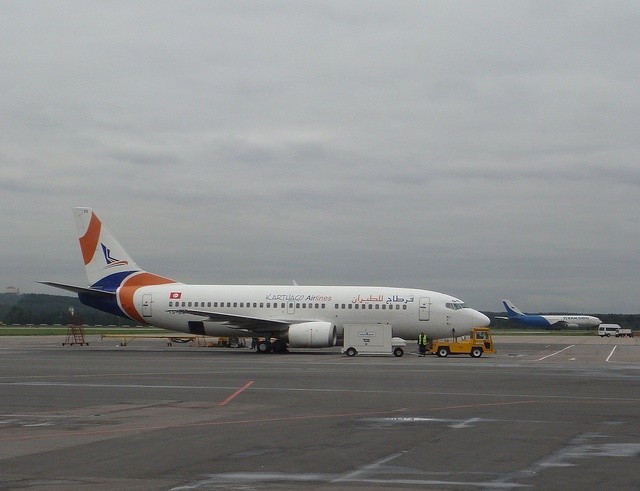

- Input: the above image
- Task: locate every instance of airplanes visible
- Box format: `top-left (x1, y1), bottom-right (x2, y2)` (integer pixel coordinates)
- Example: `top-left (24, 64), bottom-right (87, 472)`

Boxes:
top-left (494, 299), bottom-right (602, 331)
top-left (36, 205), bottom-right (491, 354)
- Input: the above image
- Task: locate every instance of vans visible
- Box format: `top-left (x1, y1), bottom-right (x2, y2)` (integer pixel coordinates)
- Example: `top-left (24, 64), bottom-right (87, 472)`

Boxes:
top-left (599, 324), bottom-right (622, 338)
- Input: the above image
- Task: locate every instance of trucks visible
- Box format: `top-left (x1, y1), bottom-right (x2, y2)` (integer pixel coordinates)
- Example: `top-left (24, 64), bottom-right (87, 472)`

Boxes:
top-left (431, 327), bottom-right (494, 358)
top-left (616, 329), bottom-right (632, 337)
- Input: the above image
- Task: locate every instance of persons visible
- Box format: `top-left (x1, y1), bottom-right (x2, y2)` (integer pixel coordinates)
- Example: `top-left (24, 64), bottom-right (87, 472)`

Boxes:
top-left (417, 333), bottom-right (429, 357)
